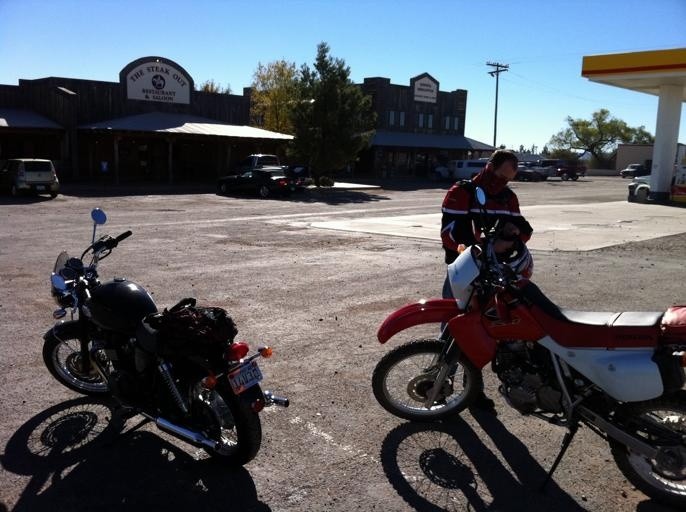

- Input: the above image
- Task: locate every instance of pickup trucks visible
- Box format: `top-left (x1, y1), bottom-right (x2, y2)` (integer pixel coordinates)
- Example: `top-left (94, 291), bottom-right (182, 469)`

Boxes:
top-left (620, 164), bottom-right (648, 179)
top-left (528, 159), bottom-right (587, 181)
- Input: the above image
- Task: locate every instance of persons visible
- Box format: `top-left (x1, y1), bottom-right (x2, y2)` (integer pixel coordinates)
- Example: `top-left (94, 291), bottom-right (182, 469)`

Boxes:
top-left (441, 149), bottom-right (533, 408)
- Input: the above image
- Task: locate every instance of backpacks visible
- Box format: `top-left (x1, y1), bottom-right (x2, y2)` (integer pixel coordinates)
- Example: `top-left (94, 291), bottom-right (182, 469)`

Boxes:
top-left (141, 296), bottom-right (238, 372)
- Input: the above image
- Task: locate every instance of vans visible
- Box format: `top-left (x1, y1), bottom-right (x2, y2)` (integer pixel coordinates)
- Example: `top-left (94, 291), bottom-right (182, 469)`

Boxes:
top-left (235, 153), bottom-right (281, 167)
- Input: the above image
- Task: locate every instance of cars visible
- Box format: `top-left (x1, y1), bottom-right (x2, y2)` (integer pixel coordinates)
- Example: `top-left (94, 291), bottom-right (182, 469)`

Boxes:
top-left (435, 158), bottom-right (537, 181)
top-left (0, 158), bottom-right (60, 198)
top-left (216, 169), bottom-right (306, 199)
top-left (628, 164), bottom-right (686, 205)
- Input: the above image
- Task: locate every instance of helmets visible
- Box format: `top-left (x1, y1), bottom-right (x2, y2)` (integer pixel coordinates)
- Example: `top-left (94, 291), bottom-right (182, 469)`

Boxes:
top-left (502, 239), bottom-right (534, 280)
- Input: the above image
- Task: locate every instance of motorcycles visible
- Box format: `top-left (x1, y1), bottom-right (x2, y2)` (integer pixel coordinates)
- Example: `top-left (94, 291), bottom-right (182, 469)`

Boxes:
top-left (42, 206), bottom-right (290, 465)
top-left (372, 186), bottom-right (686, 506)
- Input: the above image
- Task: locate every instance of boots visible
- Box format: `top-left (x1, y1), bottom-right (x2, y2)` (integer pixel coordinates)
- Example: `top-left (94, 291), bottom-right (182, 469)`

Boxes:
top-left (462, 373), bottom-right (495, 411)
